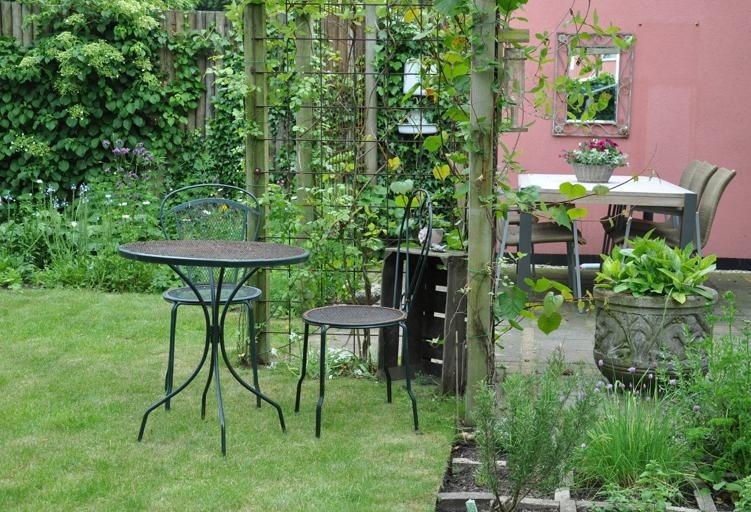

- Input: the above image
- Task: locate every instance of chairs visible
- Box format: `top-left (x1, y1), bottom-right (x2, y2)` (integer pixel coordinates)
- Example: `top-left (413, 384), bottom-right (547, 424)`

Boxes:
top-left (295, 188), bottom-right (433, 438)
top-left (158, 183), bottom-right (264, 412)
top-left (599, 158), bottom-right (738, 276)
top-left (490, 201), bottom-right (585, 314)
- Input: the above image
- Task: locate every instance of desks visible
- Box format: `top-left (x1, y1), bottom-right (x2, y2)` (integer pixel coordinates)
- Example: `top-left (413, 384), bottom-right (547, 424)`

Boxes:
top-left (517, 172), bottom-right (697, 295)
top-left (121, 241), bottom-right (310, 457)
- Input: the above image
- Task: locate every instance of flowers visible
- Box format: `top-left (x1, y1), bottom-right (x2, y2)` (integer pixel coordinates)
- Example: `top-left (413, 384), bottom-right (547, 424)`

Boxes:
top-left (557, 137), bottom-right (631, 168)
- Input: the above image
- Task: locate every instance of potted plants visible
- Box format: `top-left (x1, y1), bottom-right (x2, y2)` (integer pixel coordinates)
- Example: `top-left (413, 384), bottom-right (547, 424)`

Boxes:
top-left (592, 225), bottom-right (720, 396)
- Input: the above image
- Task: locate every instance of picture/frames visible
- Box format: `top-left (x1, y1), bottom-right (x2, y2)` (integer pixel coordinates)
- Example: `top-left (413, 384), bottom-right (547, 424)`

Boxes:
top-left (554, 32), bottom-right (634, 136)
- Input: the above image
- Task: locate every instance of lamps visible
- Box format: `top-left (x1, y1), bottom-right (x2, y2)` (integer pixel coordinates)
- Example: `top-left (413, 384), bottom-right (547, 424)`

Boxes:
top-left (493, 28), bottom-right (528, 133)
